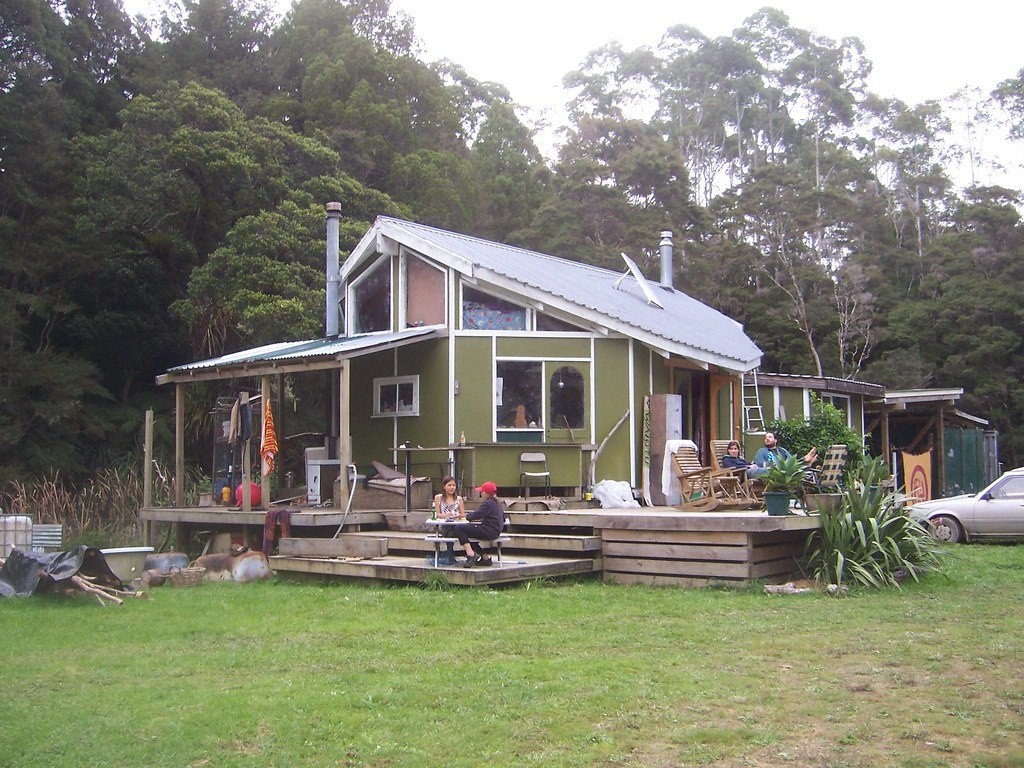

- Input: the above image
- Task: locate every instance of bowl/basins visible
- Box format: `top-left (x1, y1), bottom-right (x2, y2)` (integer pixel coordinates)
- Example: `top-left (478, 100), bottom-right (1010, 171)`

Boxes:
top-left (99, 546), bottom-right (154, 583)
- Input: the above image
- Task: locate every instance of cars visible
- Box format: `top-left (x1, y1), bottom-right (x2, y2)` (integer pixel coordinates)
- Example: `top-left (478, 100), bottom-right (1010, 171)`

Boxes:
top-left (908, 467), bottom-right (1024, 543)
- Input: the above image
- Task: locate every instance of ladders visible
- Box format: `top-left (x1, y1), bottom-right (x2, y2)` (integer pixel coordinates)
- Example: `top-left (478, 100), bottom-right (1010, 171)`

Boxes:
top-left (741, 370), bottom-right (766, 432)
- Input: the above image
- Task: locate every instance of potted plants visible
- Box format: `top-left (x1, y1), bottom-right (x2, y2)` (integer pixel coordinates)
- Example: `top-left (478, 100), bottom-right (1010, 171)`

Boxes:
top-left (803, 485), bottom-right (831, 511)
top-left (815, 484), bottom-right (846, 517)
top-left (755, 455), bottom-right (808, 515)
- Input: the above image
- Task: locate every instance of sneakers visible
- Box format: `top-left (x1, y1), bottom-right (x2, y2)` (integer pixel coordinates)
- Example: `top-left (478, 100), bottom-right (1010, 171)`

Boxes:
top-left (475, 557), bottom-right (493, 566)
top-left (464, 551), bottom-right (481, 568)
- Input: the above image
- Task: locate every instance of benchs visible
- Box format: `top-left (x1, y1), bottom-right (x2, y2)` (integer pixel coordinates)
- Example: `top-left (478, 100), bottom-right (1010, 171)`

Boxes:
top-left (426, 534), bottom-right (511, 569)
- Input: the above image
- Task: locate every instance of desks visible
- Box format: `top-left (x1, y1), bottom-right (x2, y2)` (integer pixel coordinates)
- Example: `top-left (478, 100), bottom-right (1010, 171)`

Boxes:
top-left (426, 518), bottom-right (510, 564)
top-left (388, 447), bottom-right (476, 512)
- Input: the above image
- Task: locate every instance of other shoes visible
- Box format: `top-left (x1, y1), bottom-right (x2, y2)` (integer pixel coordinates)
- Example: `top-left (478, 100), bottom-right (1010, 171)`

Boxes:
top-left (447, 553), bottom-right (458, 563)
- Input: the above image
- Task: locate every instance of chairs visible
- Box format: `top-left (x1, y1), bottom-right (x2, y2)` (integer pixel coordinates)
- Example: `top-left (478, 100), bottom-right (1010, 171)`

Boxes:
top-left (662, 440), bottom-right (848, 513)
top-left (518, 452), bottom-right (551, 501)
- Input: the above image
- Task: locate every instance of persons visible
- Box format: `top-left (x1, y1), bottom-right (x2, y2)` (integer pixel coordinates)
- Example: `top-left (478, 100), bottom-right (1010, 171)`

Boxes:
top-left (724, 441), bottom-right (818, 479)
top-left (435, 476), bottom-right (466, 562)
top-left (454, 481), bottom-right (503, 568)
top-left (754, 432), bottom-right (791, 467)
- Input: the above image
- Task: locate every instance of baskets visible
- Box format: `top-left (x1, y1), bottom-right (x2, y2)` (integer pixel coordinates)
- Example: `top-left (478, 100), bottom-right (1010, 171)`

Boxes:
top-left (166, 560), bottom-right (206, 588)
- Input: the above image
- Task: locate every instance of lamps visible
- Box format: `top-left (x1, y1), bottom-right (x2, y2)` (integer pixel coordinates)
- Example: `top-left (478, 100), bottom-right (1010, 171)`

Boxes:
top-left (558, 375), bottom-right (564, 389)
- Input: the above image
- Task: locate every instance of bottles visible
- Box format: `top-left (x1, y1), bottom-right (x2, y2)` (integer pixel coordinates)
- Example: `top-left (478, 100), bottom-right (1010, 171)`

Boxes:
top-left (461, 431), bottom-right (466, 447)
top-left (431, 501), bottom-right (436, 521)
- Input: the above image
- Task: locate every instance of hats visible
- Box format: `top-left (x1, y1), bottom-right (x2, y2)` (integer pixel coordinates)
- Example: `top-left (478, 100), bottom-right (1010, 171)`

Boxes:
top-left (474, 482), bottom-right (497, 494)
